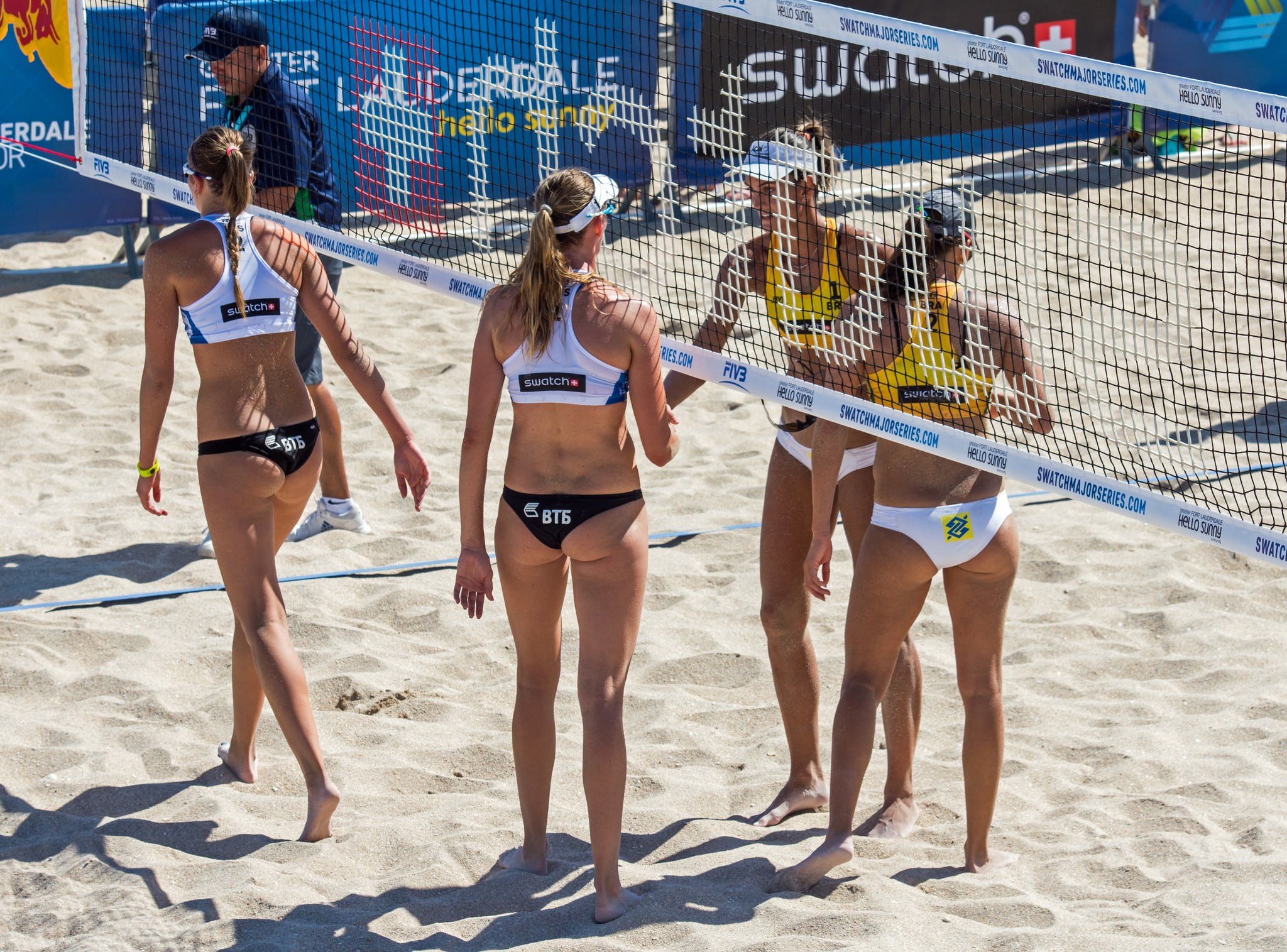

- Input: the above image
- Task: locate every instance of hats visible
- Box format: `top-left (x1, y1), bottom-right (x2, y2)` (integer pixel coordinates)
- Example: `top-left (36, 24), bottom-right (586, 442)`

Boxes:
top-left (723, 141), bottom-right (818, 182)
top-left (907, 189), bottom-right (980, 250)
top-left (183, 6), bottom-right (271, 63)
top-left (552, 169), bottom-right (619, 235)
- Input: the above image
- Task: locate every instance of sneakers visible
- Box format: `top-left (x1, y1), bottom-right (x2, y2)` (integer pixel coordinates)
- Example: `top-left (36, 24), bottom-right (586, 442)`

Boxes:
top-left (290, 495), bottom-right (372, 544)
top-left (197, 526), bottom-right (217, 559)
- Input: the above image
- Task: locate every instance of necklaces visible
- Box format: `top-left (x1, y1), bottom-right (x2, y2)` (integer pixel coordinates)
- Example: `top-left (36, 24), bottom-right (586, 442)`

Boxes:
top-left (202, 208), bottom-right (228, 216)
top-left (783, 222), bottom-right (826, 269)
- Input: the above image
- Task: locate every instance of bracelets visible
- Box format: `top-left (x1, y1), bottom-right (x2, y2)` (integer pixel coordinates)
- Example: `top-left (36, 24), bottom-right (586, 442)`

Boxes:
top-left (137, 457), bottom-right (159, 477)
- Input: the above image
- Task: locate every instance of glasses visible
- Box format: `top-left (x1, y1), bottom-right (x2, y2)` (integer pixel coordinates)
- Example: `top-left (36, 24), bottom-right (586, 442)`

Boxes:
top-left (586, 204), bottom-right (614, 218)
top-left (958, 229), bottom-right (975, 260)
top-left (182, 163), bottom-right (212, 183)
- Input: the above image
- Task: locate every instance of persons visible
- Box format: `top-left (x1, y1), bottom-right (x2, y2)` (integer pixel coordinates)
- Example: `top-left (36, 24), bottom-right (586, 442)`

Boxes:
top-left (763, 190), bottom-right (1056, 893)
top-left (665, 124), bottom-right (921, 846)
top-left (453, 168), bottom-right (679, 927)
top-left (135, 128), bottom-right (429, 842)
top-left (184, 7), bottom-right (373, 555)
